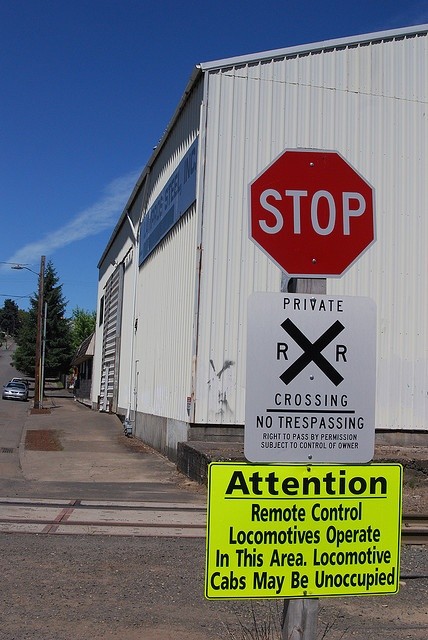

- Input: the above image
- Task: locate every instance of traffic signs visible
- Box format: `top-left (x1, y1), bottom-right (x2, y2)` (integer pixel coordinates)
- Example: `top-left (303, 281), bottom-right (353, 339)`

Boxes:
top-left (203, 459), bottom-right (405, 602)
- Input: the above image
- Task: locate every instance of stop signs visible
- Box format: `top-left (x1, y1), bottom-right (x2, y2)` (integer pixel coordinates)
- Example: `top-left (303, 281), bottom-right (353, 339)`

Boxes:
top-left (244, 291), bottom-right (380, 466)
top-left (245, 146), bottom-right (379, 281)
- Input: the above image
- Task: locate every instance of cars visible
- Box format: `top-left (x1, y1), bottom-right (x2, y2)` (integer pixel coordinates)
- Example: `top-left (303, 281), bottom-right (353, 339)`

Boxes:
top-left (2, 382), bottom-right (30, 403)
top-left (9, 378), bottom-right (33, 390)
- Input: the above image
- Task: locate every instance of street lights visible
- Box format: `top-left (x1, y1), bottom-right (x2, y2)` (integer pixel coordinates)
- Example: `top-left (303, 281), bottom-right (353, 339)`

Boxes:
top-left (11, 264), bottom-right (49, 410)
